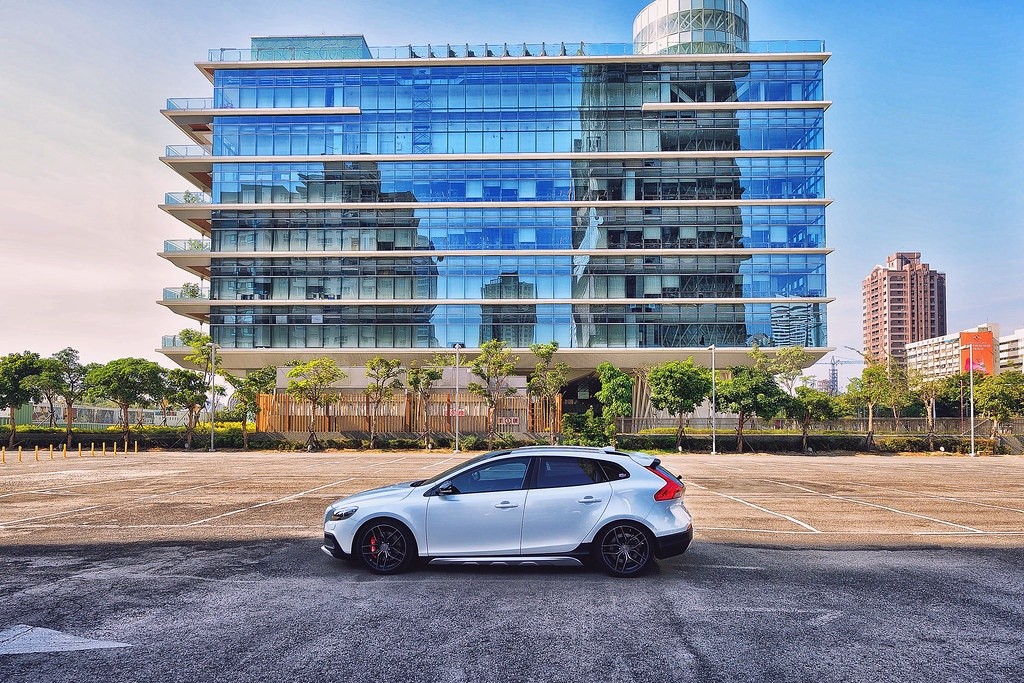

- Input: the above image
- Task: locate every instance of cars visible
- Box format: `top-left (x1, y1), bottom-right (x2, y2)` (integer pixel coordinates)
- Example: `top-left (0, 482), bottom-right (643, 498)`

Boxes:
top-left (323, 444), bottom-right (694, 580)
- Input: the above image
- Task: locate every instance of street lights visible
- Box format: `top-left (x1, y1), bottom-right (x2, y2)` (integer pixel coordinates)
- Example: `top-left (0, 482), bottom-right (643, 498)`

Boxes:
top-left (455, 343), bottom-right (462, 452)
top-left (708, 345), bottom-right (717, 455)
top-left (958, 343), bottom-right (976, 460)
top-left (206, 343), bottom-right (216, 451)
